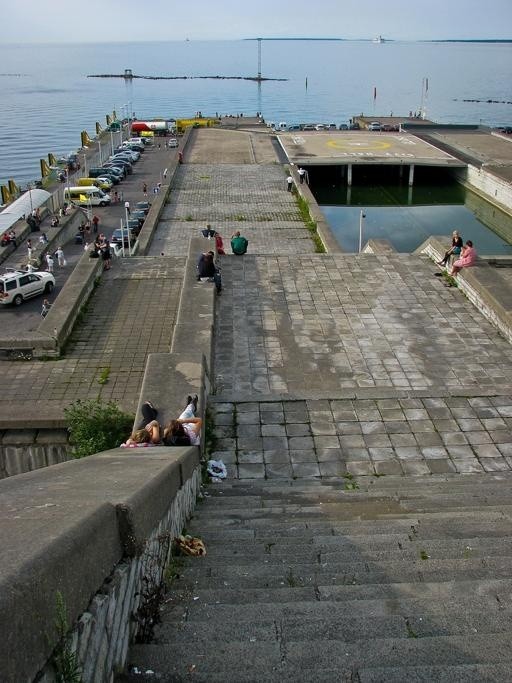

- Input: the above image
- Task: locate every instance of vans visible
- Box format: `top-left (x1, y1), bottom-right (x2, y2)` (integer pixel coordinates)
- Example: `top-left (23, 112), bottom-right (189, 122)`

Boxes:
top-left (64, 186), bottom-right (112, 208)
top-left (503, 126), bottom-right (512, 134)
top-left (78, 177), bottom-right (111, 193)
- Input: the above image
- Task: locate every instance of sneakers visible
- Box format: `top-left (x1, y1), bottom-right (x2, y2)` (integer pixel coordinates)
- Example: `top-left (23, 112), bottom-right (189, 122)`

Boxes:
top-left (186, 394), bottom-right (199, 412)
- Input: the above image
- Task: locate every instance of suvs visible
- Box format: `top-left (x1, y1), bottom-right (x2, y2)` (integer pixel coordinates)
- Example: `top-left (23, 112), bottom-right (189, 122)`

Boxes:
top-left (66, 156), bottom-right (80, 171)
top-left (168, 138), bottom-right (178, 148)
top-left (0, 270), bottom-right (57, 307)
top-left (368, 121), bottom-right (381, 132)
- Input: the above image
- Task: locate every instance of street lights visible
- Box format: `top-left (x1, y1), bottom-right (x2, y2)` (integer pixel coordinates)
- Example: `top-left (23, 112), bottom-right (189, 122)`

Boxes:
top-left (124, 201), bottom-right (132, 257)
top-left (125, 104), bottom-right (131, 136)
top-left (120, 106), bottom-right (125, 118)
top-left (129, 101), bottom-right (134, 119)
top-left (358, 207), bottom-right (369, 252)
top-left (83, 153), bottom-right (88, 177)
top-left (61, 168), bottom-right (71, 204)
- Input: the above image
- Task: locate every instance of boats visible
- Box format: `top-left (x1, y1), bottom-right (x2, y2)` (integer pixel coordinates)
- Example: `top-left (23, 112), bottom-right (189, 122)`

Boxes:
top-left (372, 34), bottom-right (385, 44)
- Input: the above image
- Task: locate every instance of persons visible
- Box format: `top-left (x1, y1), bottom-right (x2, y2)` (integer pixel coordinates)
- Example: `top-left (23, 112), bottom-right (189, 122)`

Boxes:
top-left (447, 240), bottom-right (476, 278)
top-left (197, 225), bottom-right (248, 293)
top-left (0, 141), bottom-right (183, 319)
top-left (298, 168), bottom-right (307, 184)
top-left (436, 231), bottom-right (463, 267)
top-left (286, 175), bottom-right (294, 192)
top-left (164, 393), bottom-right (203, 447)
top-left (126, 401), bottom-right (160, 444)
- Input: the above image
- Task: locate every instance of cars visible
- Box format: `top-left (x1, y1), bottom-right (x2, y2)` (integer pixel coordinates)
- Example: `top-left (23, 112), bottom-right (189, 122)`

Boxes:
top-left (135, 201), bottom-right (152, 214)
top-left (278, 122), bottom-right (338, 131)
top-left (89, 136), bottom-right (152, 185)
top-left (351, 124), bottom-right (360, 130)
top-left (130, 211), bottom-right (146, 221)
top-left (123, 219), bottom-right (142, 235)
top-left (339, 123), bottom-right (348, 130)
top-left (106, 118), bottom-right (130, 132)
top-left (111, 228), bottom-right (134, 248)
top-left (109, 242), bottom-right (123, 259)
top-left (384, 125), bottom-right (392, 132)
